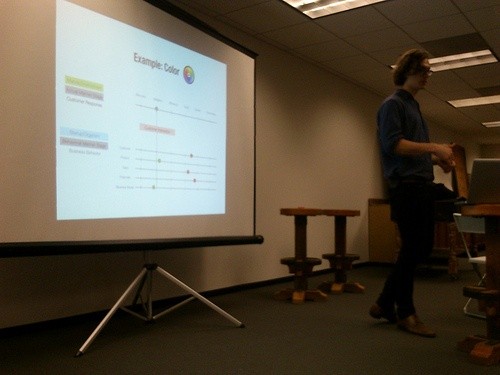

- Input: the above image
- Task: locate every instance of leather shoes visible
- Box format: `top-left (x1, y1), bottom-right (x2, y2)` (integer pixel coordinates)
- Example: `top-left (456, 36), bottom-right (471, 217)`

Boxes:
top-left (369, 303), bottom-right (397, 323)
top-left (397, 315), bottom-right (436, 337)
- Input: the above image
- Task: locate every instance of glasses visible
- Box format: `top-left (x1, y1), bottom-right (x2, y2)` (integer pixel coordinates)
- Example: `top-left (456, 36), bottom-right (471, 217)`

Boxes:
top-left (416, 67), bottom-right (434, 77)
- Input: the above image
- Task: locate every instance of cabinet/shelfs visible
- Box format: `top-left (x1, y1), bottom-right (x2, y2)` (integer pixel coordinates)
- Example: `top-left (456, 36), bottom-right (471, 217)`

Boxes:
top-left (367, 198), bottom-right (401, 265)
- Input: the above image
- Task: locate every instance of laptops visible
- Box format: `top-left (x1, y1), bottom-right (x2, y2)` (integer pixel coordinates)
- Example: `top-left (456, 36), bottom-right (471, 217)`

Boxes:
top-left (466, 158), bottom-right (500, 204)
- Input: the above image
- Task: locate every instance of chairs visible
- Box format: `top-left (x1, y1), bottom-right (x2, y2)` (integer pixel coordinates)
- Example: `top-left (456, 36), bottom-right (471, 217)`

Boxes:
top-left (453, 211), bottom-right (488, 320)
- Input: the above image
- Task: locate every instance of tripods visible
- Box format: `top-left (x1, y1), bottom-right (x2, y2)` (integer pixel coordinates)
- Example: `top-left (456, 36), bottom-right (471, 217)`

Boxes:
top-left (74, 250), bottom-right (246, 357)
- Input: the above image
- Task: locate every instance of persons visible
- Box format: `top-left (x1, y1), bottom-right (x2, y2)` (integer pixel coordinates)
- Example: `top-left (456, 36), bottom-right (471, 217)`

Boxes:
top-left (366, 48), bottom-right (456, 338)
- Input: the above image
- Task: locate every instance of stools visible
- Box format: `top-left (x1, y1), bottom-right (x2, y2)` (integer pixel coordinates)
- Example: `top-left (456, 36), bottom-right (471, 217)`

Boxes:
top-left (320, 208), bottom-right (365, 295)
top-left (279, 206), bottom-right (328, 304)
